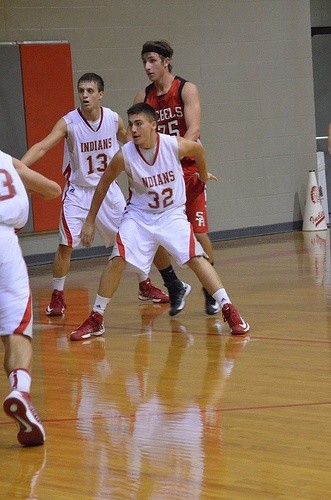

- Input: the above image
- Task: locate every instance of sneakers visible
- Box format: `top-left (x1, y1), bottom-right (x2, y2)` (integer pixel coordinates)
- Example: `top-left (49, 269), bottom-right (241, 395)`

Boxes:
top-left (225, 334), bottom-right (249, 356)
top-left (164, 281), bottom-right (191, 316)
top-left (206, 317), bottom-right (221, 336)
top-left (169, 319), bottom-right (195, 348)
top-left (204, 288), bottom-right (221, 315)
top-left (72, 338), bottom-right (105, 361)
top-left (2, 391), bottom-right (45, 446)
top-left (222, 304), bottom-right (250, 335)
top-left (137, 278), bottom-right (169, 303)
top-left (48, 314), bottom-right (65, 335)
top-left (139, 304), bottom-right (170, 318)
top-left (45, 291), bottom-right (66, 316)
top-left (12, 446), bottom-right (47, 500)
top-left (70, 311), bottom-right (105, 340)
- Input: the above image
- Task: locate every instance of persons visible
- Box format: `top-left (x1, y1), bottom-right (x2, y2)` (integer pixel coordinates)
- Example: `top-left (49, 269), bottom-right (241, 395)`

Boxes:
top-left (0, 151), bottom-right (62, 445)
top-left (124, 41), bottom-right (222, 316)
top-left (20, 72), bottom-right (169, 317)
top-left (69, 102), bottom-right (250, 340)
